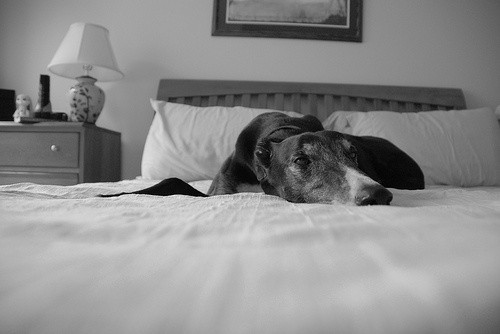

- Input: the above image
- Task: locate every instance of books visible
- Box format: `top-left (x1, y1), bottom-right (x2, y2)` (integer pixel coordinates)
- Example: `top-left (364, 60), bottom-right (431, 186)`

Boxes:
top-left (18, 116), bottom-right (53, 122)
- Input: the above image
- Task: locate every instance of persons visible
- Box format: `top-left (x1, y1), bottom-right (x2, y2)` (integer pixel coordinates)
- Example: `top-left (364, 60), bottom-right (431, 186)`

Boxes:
top-left (13, 95), bottom-right (31, 122)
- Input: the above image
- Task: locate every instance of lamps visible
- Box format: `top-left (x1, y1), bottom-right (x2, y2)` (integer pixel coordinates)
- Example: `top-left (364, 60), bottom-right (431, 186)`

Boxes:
top-left (46, 20), bottom-right (123, 126)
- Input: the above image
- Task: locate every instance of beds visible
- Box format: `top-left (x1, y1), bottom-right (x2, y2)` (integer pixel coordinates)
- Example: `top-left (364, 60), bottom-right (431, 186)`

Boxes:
top-left (0, 76), bottom-right (500, 334)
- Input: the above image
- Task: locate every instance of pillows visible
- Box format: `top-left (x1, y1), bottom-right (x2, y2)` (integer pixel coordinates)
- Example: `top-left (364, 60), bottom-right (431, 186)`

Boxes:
top-left (320, 106), bottom-right (500, 189)
top-left (139, 96), bottom-right (307, 184)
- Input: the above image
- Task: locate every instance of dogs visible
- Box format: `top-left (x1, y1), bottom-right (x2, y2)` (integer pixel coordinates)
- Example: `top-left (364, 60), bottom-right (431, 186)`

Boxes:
top-left (210, 110), bottom-right (425, 206)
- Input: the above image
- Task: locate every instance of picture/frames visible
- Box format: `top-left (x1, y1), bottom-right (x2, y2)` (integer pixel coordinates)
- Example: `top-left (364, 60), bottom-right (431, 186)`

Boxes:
top-left (211, 0), bottom-right (363, 43)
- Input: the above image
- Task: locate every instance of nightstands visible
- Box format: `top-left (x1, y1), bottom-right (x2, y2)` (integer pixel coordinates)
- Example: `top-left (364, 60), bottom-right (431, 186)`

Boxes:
top-left (0, 121), bottom-right (121, 186)
